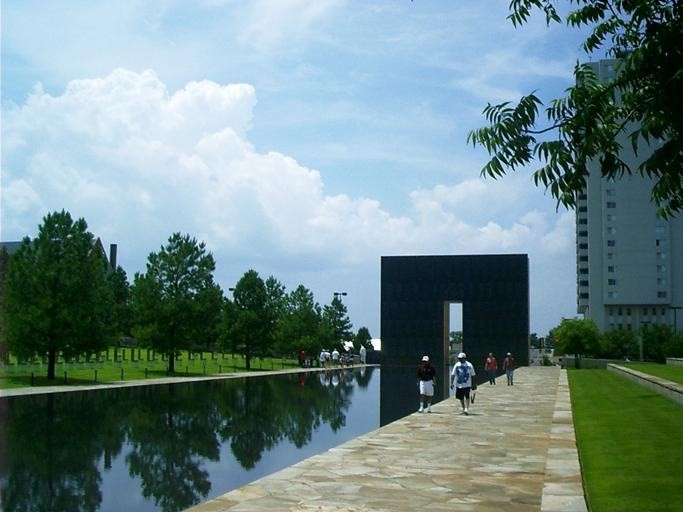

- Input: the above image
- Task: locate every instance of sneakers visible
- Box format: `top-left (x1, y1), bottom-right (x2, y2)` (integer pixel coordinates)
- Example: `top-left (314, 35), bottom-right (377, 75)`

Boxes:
top-left (459, 408), bottom-right (470, 416)
top-left (417, 407), bottom-right (431, 412)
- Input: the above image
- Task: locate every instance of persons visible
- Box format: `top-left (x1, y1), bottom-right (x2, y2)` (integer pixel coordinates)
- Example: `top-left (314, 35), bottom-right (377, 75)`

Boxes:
top-left (483, 352), bottom-right (498, 385)
top-left (502, 352), bottom-right (515, 385)
top-left (448, 352), bottom-right (476, 416)
top-left (414, 355), bottom-right (436, 413)
top-left (299, 368), bottom-right (353, 389)
top-left (299, 348), bottom-right (354, 370)
top-left (359, 345), bottom-right (366, 364)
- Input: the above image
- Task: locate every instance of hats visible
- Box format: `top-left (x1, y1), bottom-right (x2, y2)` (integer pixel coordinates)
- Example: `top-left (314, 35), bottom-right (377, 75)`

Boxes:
top-left (421, 355), bottom-right (429, 361)
top-left (457, 352), bottom-right (466, 359)
